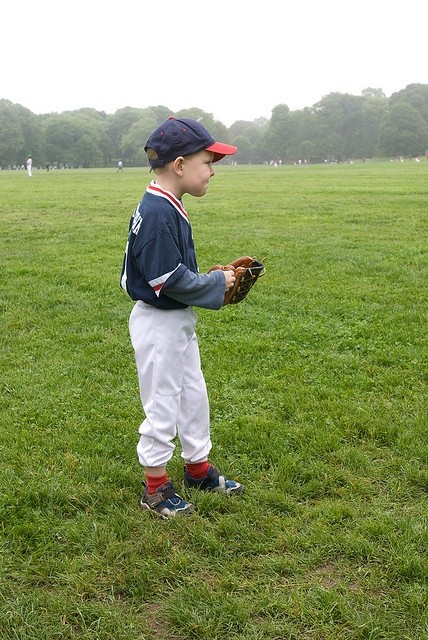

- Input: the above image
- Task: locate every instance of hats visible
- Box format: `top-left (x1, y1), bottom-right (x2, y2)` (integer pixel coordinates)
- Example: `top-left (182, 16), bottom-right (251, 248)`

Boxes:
top-left (144, 116), bottom-right (238, 174)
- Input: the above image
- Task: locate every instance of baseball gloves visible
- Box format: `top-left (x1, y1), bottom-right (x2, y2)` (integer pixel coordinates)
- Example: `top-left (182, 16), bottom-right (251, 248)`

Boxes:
top-left (208, 254), bottom-right (269, 306)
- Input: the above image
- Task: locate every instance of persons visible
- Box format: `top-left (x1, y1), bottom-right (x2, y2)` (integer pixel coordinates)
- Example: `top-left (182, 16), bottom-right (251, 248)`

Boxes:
top-left (117, 160), bottom-right (123, 174)
top-left (24, 154), bottom-right (33, 177)
top-left (118, 116), bottom-right (249, 522)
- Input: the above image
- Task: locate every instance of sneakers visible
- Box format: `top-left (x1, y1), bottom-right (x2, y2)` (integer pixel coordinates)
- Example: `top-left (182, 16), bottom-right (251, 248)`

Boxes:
top-left (140, 481), bottom-right (198, 520)
top-left (184, 464), bottom-right (244, 497)
